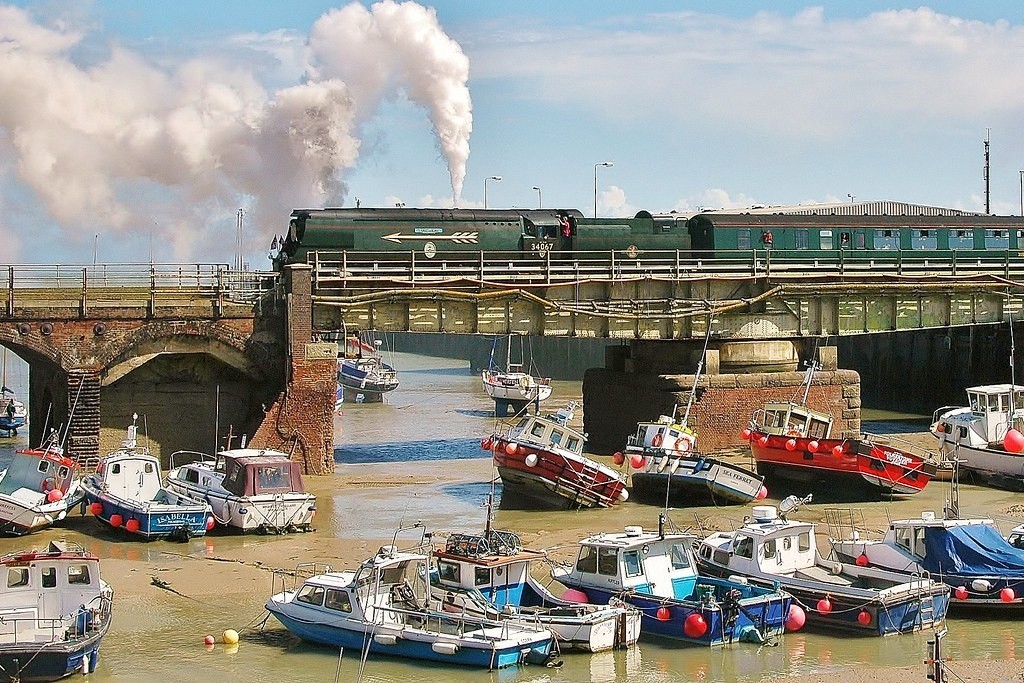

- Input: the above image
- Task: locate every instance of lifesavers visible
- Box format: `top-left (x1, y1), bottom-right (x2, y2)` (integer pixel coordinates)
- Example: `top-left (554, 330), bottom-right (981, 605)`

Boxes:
top-left (675, 437), bottom-right (692, 456)
top-left (787, 430), bottom-right (802, 437)
top-left (488, 378), bottom-right (494, 383)
top-left (652, 434), bottom-right (663, 447)
top-left (43, 477), bottom-right (59, 494)
top-left (520, 377), bottom-right (530, 386)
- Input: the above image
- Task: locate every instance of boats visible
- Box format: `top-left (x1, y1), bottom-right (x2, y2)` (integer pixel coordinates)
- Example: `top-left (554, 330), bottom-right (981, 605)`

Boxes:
top-left (825, 507), bottom-right (1024, 612)
top-left (0, 426), bottom-right (86, 536)
top-left (613, 359), bottom-right (765, 504)
top-left (337, 331), bottom-right (400, 393)
top-left (267, 523), bottom-right (559, 670)
top-left (749, 360), bottom-right (935, 497)
top-left (420, 495), bottom-right (644, 652)
top-left (1, 540), bottom-right (113, 682)
top-left (695, 503), bottom-right (951, 635)
top-left (163, 423), bottom-right (316, 530)
top-left (335, 383), bottom-right (343, 414)
top-left (929, 383), bottom-right (1024, 480)
top-left (80, 415), bottom-right (211, 539)
top-left (481, 335), bottom-right (553, 416)
top-left (482, 399), bottom-right (628, 510)
top-left (548, 526), bottom-right (793, 647)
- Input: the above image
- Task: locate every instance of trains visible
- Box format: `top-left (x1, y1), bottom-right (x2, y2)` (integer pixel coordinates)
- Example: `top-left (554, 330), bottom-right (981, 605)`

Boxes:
top-left (271, 208), bottom-right (1024, 273)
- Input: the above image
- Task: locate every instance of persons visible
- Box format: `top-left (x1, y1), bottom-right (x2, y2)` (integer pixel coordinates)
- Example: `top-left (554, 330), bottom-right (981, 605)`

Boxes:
top-left (559, 216), bottom-right (572, 237)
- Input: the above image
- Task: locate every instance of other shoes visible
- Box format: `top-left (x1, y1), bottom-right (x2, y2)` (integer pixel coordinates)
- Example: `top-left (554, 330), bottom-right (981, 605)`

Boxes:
top-left (12, 434), bottom-right (17, 436)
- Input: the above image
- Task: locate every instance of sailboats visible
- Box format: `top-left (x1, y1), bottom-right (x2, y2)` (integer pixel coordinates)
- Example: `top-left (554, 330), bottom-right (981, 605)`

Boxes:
top-left (0, 346), bottom-right (27, 433)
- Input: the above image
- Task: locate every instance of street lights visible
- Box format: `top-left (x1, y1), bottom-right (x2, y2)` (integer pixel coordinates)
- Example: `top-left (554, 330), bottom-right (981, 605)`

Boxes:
top-left (533, 187), bottom-right (541, 209)
top-left (1019, 171), bottom-right (1024, 216)
top-left (485, 176), bottom-right (502, 210)
top-left (595, 162), bottom-right (614, 219)
